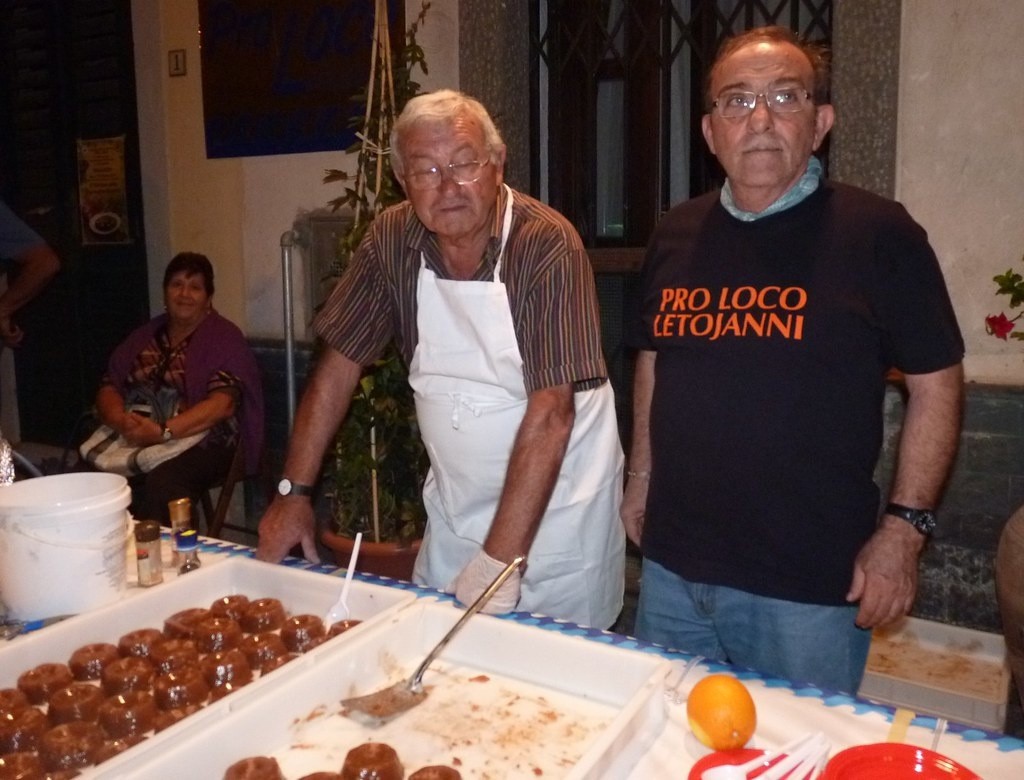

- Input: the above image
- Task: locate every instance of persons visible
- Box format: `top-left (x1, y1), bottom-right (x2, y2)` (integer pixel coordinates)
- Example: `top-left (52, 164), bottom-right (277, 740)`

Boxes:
top-left (95, 251), bottom-right (266, 525)
top-left (998, 506), bottom-right (1024, 731)
top-left (259, 91), bottom-right (630, 641)
top-left (0, 204), bottom-right (62, 452)
top-left (615, 24), bottom-right (966, 698)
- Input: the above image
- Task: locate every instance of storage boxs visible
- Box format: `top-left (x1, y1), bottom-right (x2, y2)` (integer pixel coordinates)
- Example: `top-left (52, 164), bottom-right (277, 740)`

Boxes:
top-left (0, 555), bottom-right (1013, 780)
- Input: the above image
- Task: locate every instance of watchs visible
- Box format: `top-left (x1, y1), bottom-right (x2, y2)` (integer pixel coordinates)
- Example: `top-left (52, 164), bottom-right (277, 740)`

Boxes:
top-left (883, 500), bottom-right (937, 535)
top-left (159, 421), bottom-right (171, 444)
top-left (272, 474), bottom-right (311, 497)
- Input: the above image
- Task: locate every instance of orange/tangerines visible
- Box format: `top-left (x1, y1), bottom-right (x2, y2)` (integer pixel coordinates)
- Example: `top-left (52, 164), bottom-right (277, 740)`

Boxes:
top-left (687, 674), bottom-right (758, 751)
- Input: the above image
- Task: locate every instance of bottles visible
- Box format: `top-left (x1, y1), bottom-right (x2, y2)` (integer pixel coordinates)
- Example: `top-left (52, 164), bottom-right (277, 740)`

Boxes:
top-left (174, 529), bottom-right (202, 576)
top-left (134, 520), bottom-right (164, 587)
top-left (168, 496), bottom-right (191, 564)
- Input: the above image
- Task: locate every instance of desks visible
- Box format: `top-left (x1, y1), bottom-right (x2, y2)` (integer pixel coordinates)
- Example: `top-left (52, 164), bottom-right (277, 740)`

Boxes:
top-left (0, 520), bottom-right (1024, 780)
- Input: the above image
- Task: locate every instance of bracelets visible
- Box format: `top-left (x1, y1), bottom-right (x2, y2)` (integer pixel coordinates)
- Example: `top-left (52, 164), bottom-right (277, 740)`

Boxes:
top-left (625, 469), bottom-right (651, 479)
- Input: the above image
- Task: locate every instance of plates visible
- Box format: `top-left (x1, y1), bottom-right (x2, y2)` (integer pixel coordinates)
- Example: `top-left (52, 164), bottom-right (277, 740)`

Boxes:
top-left (89, 212), bottom-right (121, 234)
top-left (826, 742), bottom-right (983, 780)
top-left (687, 749), bottom-right (823, 780)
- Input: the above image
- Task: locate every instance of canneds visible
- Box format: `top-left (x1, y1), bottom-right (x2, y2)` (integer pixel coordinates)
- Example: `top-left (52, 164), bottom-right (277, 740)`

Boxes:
top-left (135, 520), bottom-right (162, 587)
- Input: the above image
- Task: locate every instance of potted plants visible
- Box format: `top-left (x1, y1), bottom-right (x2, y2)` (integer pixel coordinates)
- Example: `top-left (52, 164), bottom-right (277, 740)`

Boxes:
top-left (314, 1), bottom-right (437, 578)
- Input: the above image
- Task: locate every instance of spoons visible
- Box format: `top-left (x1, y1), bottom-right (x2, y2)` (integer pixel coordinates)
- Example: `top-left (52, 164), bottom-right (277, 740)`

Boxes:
top-left (323, 532), bottom-right (362, 632)
top-left (700, 730), bottom-right (811, 780)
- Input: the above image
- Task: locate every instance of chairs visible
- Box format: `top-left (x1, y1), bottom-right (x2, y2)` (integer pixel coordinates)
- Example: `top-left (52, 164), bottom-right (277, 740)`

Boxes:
top-left (139, 347), bottom-right (263, 537)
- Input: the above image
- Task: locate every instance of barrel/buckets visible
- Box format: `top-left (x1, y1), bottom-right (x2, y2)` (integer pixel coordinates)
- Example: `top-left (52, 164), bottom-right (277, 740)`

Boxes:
top-left (0, 472), bottom-right (132, 624)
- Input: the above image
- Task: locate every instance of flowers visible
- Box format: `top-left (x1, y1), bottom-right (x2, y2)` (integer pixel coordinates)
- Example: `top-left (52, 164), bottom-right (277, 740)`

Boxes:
top-left (985, 270), bottom-right (1024, 341)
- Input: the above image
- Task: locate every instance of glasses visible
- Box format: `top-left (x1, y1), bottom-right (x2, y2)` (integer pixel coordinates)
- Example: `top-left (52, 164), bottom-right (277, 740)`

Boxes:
top-left (708, 88), bottom-right (818, 117)
top-left (401, 153), bottom-right (490, 191)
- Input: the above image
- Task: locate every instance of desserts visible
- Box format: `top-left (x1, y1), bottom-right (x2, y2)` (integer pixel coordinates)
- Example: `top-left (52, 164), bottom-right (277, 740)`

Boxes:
top-left (0, 591), bottom-right (463, 780)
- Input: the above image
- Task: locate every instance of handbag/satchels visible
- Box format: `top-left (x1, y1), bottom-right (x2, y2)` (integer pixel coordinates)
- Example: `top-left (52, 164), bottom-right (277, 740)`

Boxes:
top-left (78, 388), bottom-right (211, 478)
